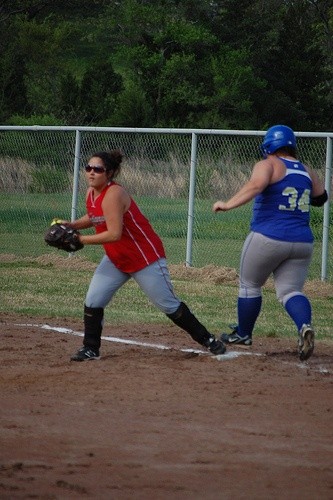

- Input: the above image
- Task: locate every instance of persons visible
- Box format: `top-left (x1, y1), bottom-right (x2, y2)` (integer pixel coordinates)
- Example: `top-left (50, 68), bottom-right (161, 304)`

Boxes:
top-left (43, 151), bottom-right (227, 364)
top-left (211, 125), bottom-right (329, 363)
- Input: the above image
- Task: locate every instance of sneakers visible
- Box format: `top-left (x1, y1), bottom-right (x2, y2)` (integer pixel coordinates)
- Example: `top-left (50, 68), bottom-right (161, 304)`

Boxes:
top-left (298, 324), bottom-right (315, 361)
top-left (207, 334), bottom-right (226, 354)
top-left (71, 346), bottom-right (100, 361)
top-left (221, 330), bottom-right (252, 345)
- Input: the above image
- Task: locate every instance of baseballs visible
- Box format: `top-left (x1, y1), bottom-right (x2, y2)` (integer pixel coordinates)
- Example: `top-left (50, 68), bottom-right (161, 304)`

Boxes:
top-left (50, 220), bottom-right (62, 227)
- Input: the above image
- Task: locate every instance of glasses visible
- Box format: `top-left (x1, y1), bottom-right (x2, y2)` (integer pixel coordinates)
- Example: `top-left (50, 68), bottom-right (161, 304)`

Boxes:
top-left (85, 165), bottom-right (107, 174)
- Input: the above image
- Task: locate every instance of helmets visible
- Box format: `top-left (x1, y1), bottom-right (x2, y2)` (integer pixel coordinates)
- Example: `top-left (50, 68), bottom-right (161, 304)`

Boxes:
top-left (260, 125), bottom-right (296, 159)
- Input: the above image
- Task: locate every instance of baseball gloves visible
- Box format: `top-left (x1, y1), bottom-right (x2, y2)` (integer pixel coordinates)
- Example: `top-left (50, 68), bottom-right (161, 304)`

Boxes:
top-left (44, 224), bottom-right (84, 254)
